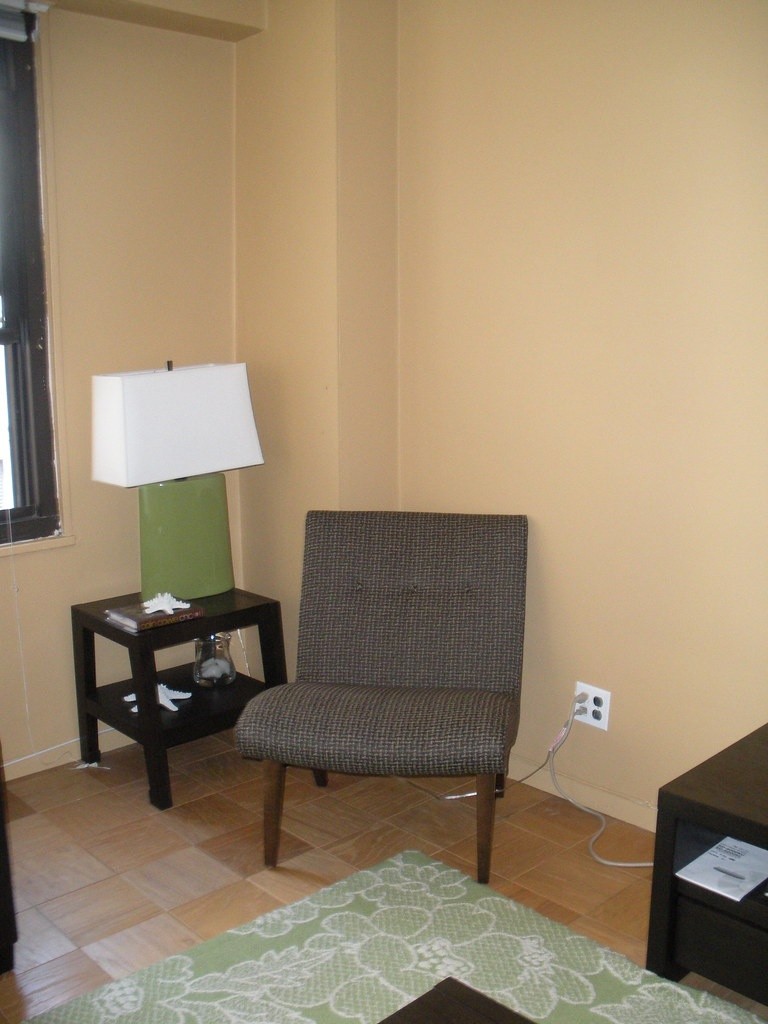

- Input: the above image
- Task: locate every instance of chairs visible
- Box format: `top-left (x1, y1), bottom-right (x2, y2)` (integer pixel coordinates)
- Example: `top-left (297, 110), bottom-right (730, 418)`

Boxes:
top-left (234, 509), bottom-right (530, 884)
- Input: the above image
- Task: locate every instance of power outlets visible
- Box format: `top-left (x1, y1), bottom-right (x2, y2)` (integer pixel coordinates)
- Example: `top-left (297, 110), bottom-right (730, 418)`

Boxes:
top-left (571, 680), bottom-right (613, 733)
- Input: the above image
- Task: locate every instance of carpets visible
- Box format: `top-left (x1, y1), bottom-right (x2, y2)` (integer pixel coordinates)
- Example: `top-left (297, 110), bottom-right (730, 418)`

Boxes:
top-left (15, 846), bottom-right (768, 1024)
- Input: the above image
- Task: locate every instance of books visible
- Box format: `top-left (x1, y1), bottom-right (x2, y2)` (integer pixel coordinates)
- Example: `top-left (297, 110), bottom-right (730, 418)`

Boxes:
top-left (674, 836), bottom-right (768, 902)
top-left (104, 592), bottom-right (205, 632)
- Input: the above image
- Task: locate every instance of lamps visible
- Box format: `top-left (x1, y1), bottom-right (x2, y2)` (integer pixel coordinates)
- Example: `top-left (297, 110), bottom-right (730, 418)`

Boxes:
top-left (91, 359), bottom-right (266, 604)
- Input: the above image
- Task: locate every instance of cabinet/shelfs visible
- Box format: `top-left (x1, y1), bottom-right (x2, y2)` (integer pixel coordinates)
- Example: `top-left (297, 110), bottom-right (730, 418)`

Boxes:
top-left (646, 721), bottom-right (768, 1010)
top-left (70, 585), bottom-right (287, 809)
